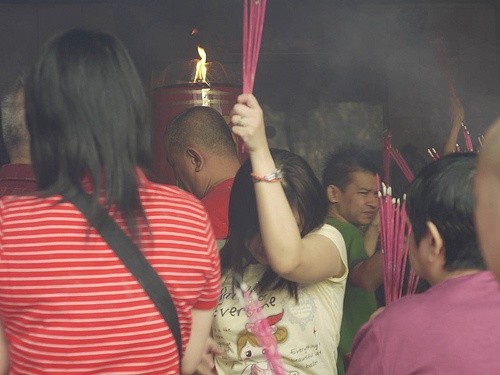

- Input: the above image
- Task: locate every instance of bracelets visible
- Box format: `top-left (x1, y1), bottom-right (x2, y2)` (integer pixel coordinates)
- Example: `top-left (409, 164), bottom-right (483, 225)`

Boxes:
top-left (249, 169), bottom-right (283, 184)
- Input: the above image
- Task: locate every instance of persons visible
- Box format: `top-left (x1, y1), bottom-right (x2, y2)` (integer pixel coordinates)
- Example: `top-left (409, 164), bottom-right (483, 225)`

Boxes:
top-left (0, 28), bottom-right (500, 375)
